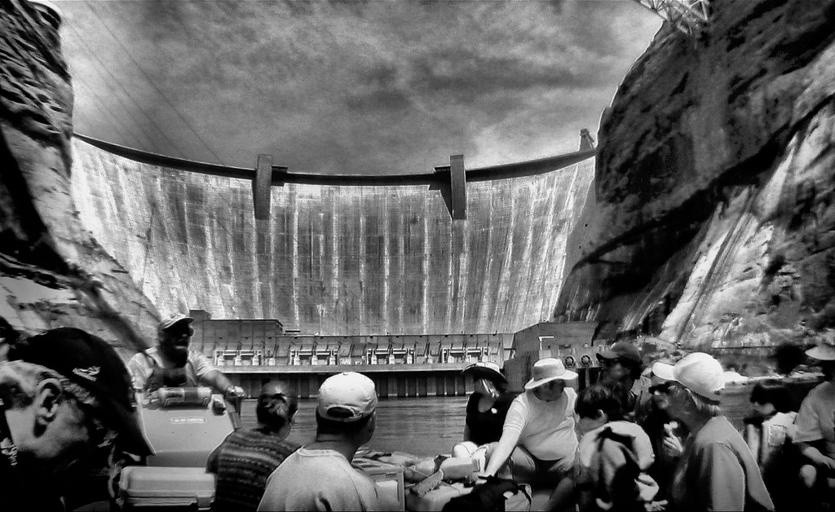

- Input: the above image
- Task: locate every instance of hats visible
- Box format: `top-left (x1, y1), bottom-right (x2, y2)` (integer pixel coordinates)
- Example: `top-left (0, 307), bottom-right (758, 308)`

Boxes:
top-left (596, 342), bottom-right (641, 362)
top-left (160, 313), bottom-right (193, 330)
top-left (652, 352), bottom-right (725, 401)
top-left (525, 358), bottom-right (579, 389)
top-left (20, 328), bottom-right (154, 456)
top-left (318, 371), bottom-right (378, 421)
top-left (463, 362), bottom-right (506, 382)
top-left (805, 330), bottom-right (835, 360)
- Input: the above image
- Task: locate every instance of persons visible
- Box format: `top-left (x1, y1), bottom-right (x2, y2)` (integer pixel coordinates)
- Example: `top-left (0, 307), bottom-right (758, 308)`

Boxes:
top-left (256, 372), bottom-right (384, 511)
top-left (453, 327), bottom-right (835, 512)
top-left (124, 310), bottom-right (244, 408)
top-left (0, 322), bottom-right (160, 512)
top-left (204, 371), bottom-right (304, 511)
